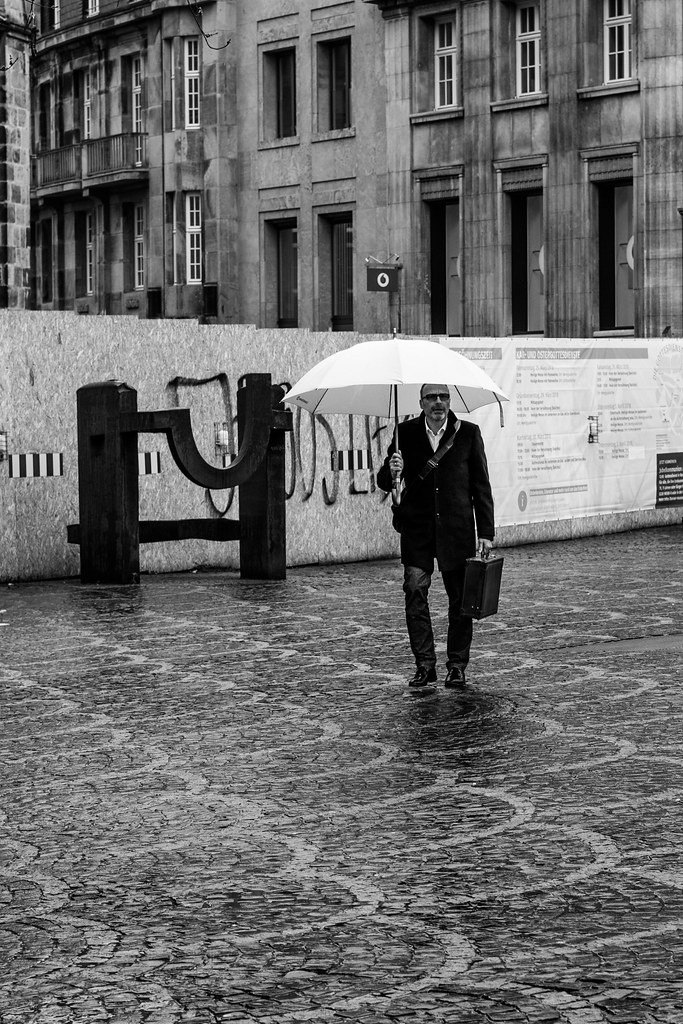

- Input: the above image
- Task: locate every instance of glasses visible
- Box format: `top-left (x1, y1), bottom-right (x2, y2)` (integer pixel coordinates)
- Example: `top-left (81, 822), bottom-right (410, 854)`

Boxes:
top-left (422, 393), bottom-right (450, 402)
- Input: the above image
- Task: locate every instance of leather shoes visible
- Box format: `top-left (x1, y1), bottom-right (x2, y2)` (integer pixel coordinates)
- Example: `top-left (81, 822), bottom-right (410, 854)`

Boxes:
top-left (445, 667), bottom-right (465, 687)
top-left (409, 666), bottom-right (438, 687)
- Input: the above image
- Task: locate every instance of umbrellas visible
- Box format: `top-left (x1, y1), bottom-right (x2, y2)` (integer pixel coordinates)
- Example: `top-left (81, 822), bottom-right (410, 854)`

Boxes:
top-left (278, 327), bottom-right (509, 506)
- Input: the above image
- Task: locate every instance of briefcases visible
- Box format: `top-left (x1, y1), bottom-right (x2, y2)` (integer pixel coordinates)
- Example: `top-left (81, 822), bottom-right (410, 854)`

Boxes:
top-left (459, 549), bottom-right (504, 620)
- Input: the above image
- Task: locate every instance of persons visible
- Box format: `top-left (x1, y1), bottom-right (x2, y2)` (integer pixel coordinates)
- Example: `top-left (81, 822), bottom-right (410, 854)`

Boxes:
top-left (375, 384), bottom-right (495, 688)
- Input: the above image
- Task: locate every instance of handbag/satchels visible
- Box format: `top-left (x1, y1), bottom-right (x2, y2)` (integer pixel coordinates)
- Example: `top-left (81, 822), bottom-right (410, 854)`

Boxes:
top-left (391, 487), bottom-right (407, 535)
